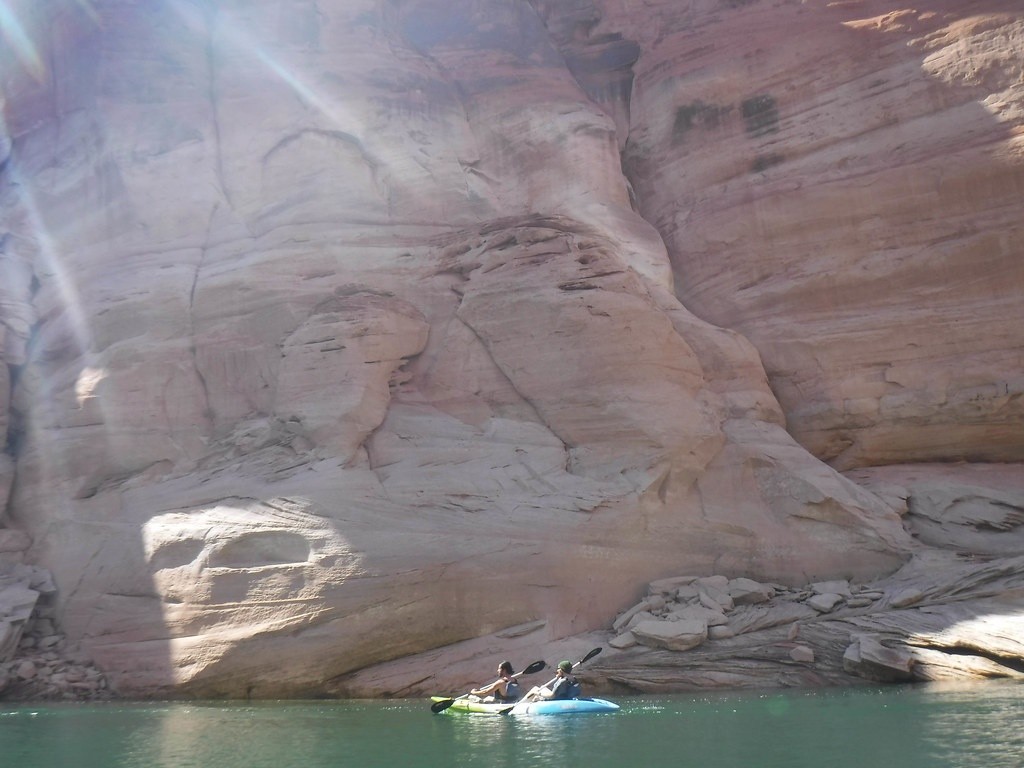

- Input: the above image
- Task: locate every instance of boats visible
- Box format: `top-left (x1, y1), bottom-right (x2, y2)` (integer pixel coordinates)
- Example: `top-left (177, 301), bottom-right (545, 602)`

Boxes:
top-left (430, 695), bottom-right (621, 716)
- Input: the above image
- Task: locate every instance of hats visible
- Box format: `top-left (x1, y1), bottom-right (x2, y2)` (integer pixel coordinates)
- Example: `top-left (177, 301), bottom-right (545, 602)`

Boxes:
top-left (559, 661), bottom-right (572, 673)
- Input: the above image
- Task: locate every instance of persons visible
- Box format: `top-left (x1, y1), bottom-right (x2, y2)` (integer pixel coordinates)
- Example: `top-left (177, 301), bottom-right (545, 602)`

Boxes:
top-left (514, 661), bottom-right (581, 705)
top-left (468, 661), bottom-right (518, 703)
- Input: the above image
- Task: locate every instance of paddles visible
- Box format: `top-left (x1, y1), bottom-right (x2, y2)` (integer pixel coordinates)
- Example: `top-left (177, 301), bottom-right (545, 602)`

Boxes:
top-left (497, 646), bottom-right (603, 714)
top-left (430, 659), bottom-right (546, 715)
